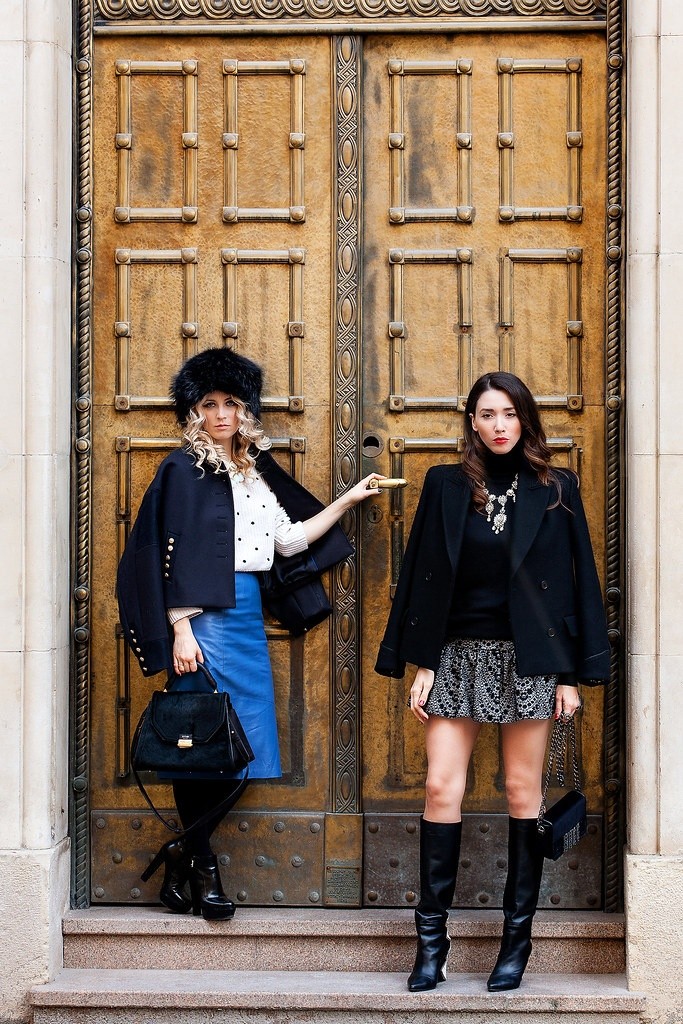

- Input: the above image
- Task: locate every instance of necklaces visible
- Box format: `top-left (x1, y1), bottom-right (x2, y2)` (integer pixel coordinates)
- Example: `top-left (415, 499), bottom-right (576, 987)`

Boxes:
top-left (481, 473), bottom-right (519, 534)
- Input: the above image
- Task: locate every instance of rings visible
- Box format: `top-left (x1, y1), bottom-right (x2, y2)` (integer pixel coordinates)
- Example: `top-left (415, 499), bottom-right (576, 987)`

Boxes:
top-left (178, 664), bottom-right (184, 667)
top-left (564, 713), bottom-right (571, 720)
top-left (576, 706), bottom-right (579, 710)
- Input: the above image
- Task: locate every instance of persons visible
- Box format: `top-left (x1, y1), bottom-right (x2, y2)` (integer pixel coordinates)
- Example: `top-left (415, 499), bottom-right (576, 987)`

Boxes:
top-left (373, 371), bottom-right (611, 993)
top-left (115, 348), bottom-right (388, 921)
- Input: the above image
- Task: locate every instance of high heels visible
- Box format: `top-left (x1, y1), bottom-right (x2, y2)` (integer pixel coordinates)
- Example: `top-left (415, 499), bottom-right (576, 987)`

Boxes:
top-left (187, 852), bottom-right (236, 921)
top-left (140, 835), bottom-right (192, 914)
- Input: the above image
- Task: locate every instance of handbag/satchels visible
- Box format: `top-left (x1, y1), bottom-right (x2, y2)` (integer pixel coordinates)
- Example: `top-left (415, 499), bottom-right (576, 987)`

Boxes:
top-left (537, 711), bottom-right (587, 861)
top-left (128, 661), bottom-right (255, 834)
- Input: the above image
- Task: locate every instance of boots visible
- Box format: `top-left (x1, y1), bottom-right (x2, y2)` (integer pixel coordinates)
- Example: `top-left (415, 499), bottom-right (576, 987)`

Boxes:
top-left (487, 815), bottom-right (544, 995)
top-left (407, 815), bottom-right (462, 992)
